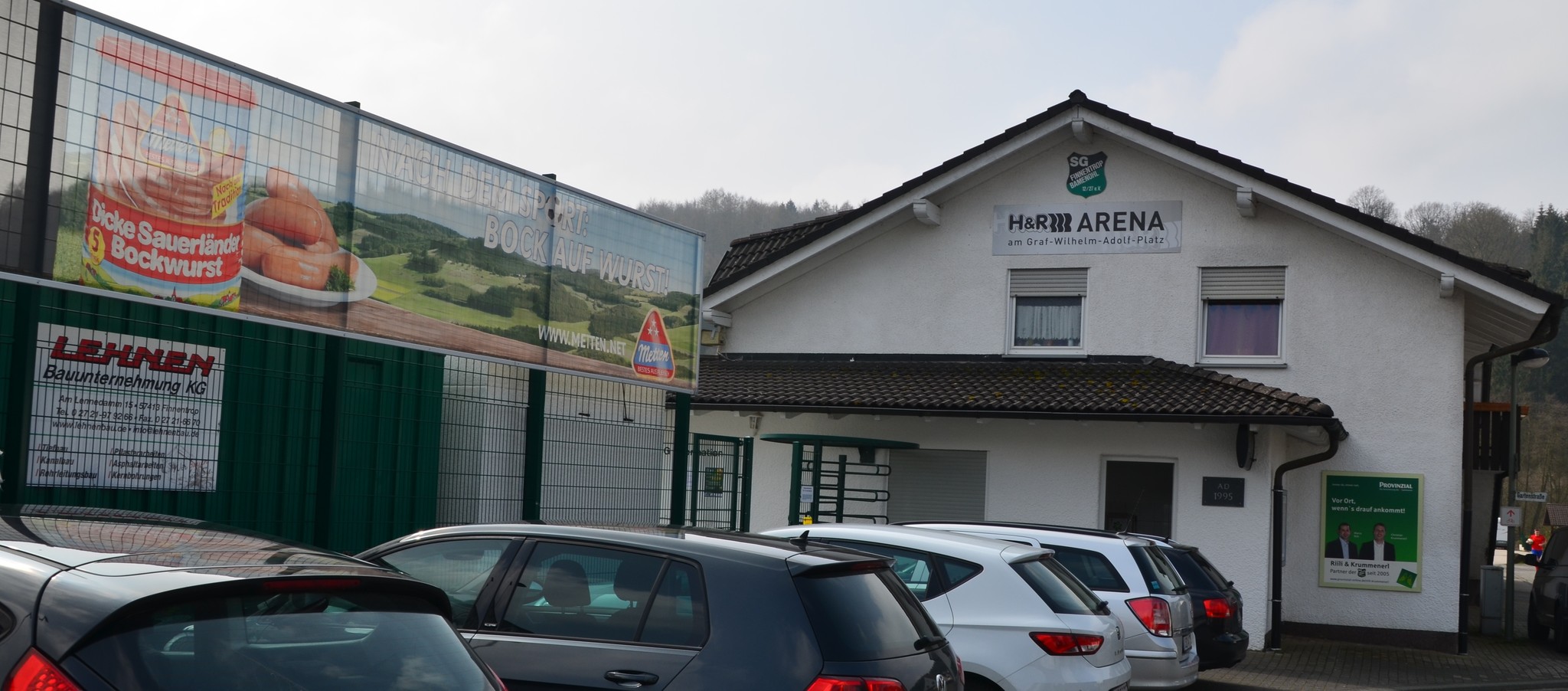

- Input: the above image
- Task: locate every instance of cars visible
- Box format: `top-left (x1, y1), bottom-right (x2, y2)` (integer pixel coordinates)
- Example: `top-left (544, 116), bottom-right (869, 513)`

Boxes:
top-left (0, 503), bottom-right (508, 690)
top-left (355, 521), bottom-right (974, 690)
top-left (533, 524), bottom-right (1136, 690)
top-left (1520, 525), bottom-right (1568, 651)
top-left (890, 524), bottom-right (1252, 690)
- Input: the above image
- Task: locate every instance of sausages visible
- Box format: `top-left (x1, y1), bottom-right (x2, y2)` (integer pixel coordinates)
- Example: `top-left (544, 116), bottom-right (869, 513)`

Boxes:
top-left (241, 222), bottom-right (332, 271)
top-left (261, 245), bottom-right (358, 289)
top-left (266, 165), bottom-right (339, 255)
top-left (241, 195), bottom-right (323, 245)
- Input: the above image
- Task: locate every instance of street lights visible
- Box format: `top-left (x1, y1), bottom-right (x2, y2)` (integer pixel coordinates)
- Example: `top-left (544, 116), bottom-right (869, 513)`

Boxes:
top-left (1503, 346), bottom-right (1553, 637)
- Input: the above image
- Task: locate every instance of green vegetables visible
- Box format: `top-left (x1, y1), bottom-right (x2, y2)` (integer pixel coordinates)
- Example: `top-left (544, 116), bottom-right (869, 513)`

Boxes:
top-left (324, 265), bottom-right (357, 293)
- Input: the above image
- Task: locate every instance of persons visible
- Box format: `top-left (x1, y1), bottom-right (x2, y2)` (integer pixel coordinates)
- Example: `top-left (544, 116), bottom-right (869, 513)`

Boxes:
top-left (1526, 529), bottom-right (1547, 571)
top-left (1359, 523), bottom-right (1395, 561)
top-left (1324, 522), bottom-right (1358, 560)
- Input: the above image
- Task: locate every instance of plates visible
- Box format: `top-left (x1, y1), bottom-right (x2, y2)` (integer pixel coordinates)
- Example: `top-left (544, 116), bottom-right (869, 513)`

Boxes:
top-left (240, 246), bottom-right (378, 308)
